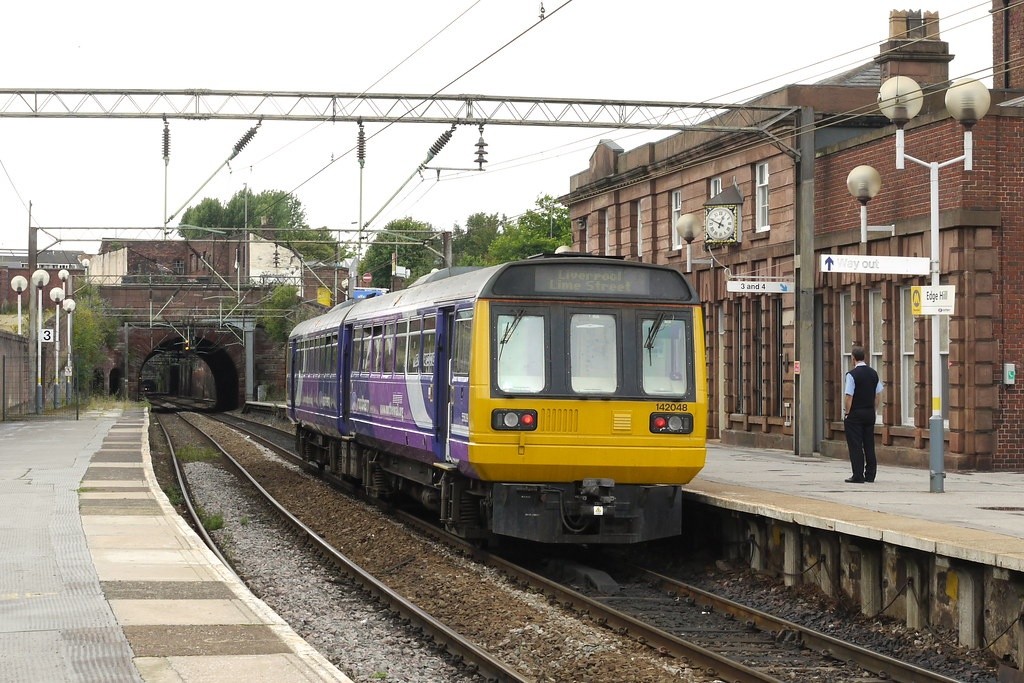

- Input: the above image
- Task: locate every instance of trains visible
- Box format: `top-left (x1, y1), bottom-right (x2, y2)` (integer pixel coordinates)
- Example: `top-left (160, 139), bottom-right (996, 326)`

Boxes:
top-left (281, 248), bottom-right (710, 555)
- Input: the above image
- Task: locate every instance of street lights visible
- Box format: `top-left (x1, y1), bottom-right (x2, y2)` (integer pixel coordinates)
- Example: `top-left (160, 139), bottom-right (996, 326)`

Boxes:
top-left (33, 269), bottom-right (51, 417)
top-left (63, 299), bottom-right (76, 409)
top-left (49, 287), bottom-right (64, 411)
top-left (9, 274), bottom-right (30, 336)
top-left (877, 76), bottom-right (994, 492)
top-left (58, 269), bottom-right (70, 304)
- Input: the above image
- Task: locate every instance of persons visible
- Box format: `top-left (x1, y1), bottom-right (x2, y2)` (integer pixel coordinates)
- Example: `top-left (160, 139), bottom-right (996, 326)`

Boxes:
top-left (840, 347), bottom-right (885, 483)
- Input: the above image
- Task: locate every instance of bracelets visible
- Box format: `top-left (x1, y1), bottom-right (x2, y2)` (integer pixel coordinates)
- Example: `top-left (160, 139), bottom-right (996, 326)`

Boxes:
top-left (844, 413), bottom-right (849, 415)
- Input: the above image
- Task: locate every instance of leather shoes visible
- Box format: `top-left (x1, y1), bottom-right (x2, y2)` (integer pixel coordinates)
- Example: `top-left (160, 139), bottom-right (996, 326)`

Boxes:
top-left (845, 477), bottom-right (864, 483)
top-left (862, 476), bottom-right (874, 482)
